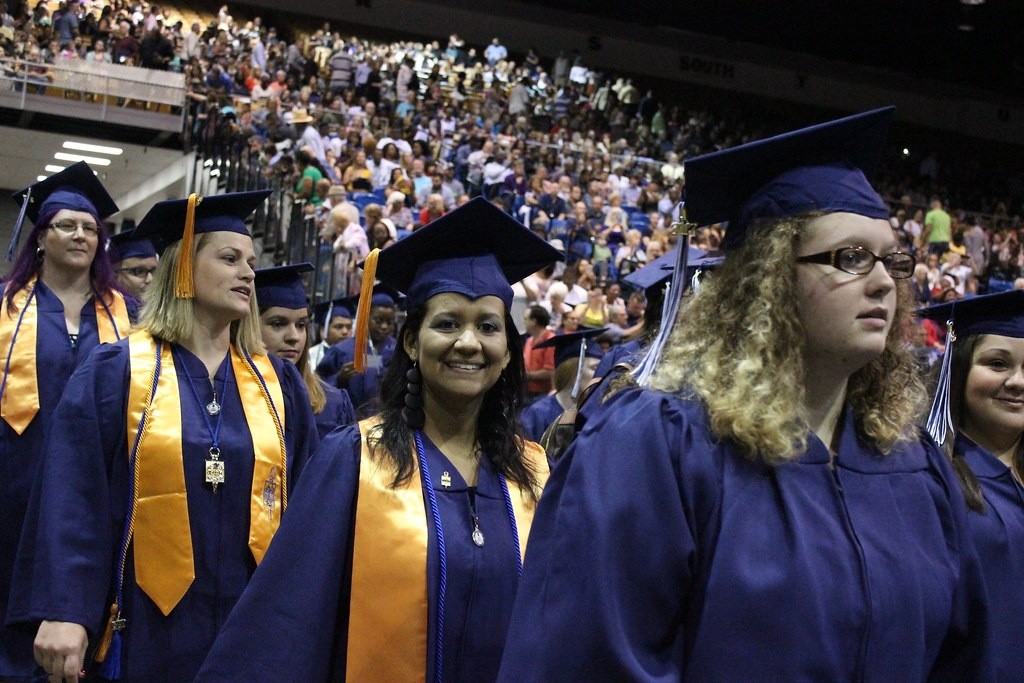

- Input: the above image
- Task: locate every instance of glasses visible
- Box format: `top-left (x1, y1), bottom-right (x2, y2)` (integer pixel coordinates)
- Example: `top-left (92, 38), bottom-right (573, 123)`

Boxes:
top-left (115, 267), bottom-right (156, 278)
top-left (44, 219), bottom-right (101, 237)
top-left (792, 247), bottom-right (916, 278)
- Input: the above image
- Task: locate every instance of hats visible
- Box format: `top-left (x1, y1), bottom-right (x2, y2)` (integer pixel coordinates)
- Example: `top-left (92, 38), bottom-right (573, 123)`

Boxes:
top-left (6, 160), bottom-right (121, 261)
top-left (911, 288), bottom-right (1024, 446)
top-left (109, 230), bottom-right (156, 263)
top-left (129, 190), bottom-right (273, 298)
top-left (532, 327), bottom-right (611, 398)
top-left (618, 103), bottom-right (909, 388)
top-left (354, 196), bottom-right (566, 373)
top-left (326, 184), bottom-right (348, 195)
top-left (254, 262), bottom-right (316, 316)
top-left (314, 294), bottom-right (355, 338)
top-left (285, 108), bottom-right (314, 124)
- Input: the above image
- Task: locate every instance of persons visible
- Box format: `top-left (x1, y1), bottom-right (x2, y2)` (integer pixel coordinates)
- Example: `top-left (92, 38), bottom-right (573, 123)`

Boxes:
top-left (191, 195), bottom-right (566, 683)
top-left (304, 298), bottom-right (357, 375)
top-left (184, 3), bottom-right (751, 471)
top-left (253, 261), bottom-right (359, 444)
top-left (316, 282), bottom-right (403, 421)
top-left (908, 285), bottom-right (1023, 683)
top-left (25, 192), bottom-right (323, 683)
top-left (0, 0), bottom-right (204, 115)
top-left (106, 225), bottom-right (159, 302)
top-left (489, 101), bottom-right (998, 683)
top-left (870, 169), bottom-right (1024, 367)
top-left (0, 162), bottom-right (146, 683)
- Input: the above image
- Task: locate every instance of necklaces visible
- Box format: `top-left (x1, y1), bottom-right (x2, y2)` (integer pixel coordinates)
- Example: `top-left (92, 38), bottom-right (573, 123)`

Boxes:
top-left (177, 350), bottom-right (234, 493)
